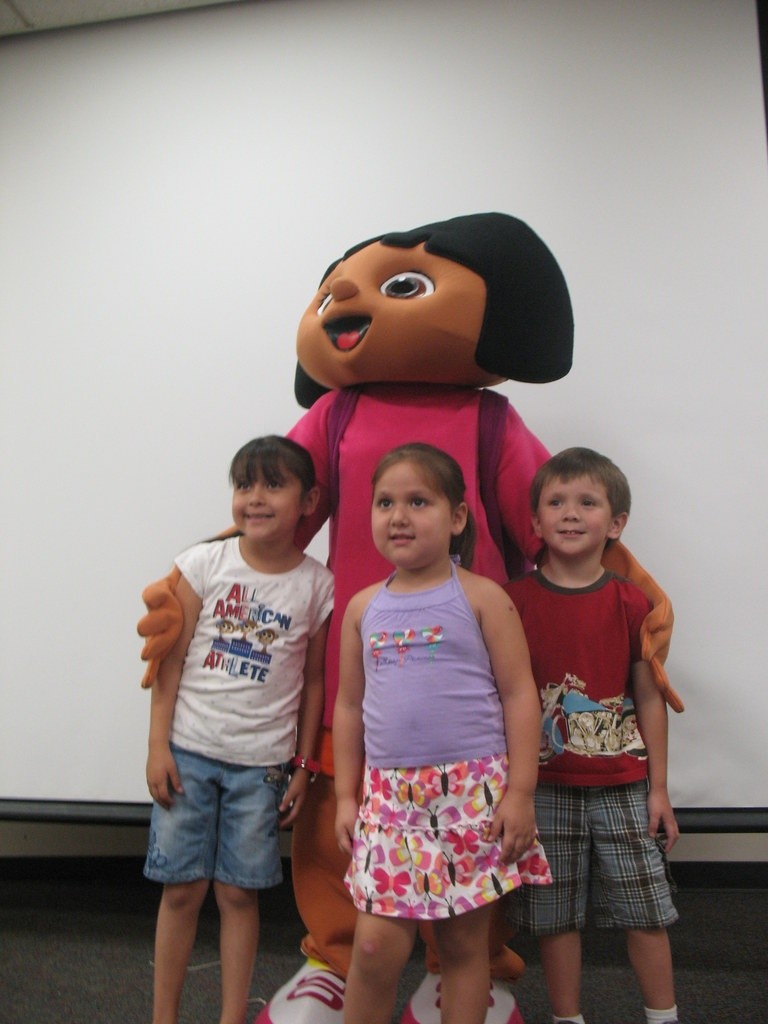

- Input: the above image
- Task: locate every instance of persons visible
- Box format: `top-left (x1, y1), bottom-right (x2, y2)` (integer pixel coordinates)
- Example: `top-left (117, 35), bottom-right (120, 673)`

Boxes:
top-left (331, 441), bottom-right (555, 1024)
top-left (143, 436), bottom-right (334, 1024)
top-left (502, 446), bottom-right (680, 1024)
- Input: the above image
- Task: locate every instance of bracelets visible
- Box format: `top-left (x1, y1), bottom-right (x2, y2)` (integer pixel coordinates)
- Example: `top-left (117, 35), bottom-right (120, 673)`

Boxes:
top-left (291, 754), bottom-right (320, 774)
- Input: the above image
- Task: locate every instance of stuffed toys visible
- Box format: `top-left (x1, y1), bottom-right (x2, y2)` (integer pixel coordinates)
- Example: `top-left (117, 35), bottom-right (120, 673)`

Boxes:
top-left (137, 212), bottom-right (684, 1024)
